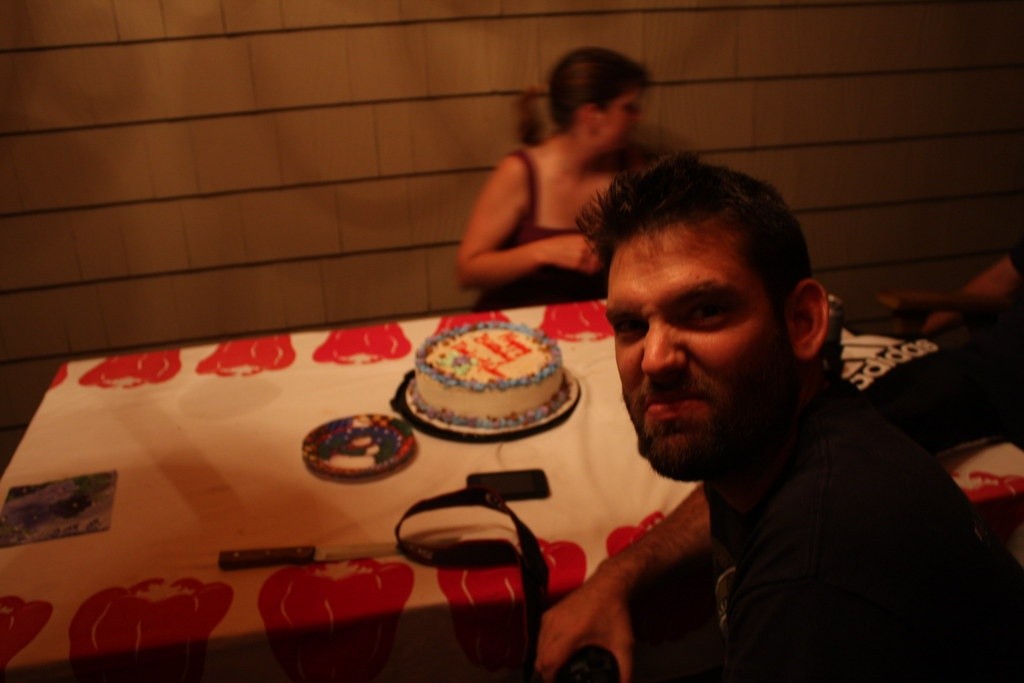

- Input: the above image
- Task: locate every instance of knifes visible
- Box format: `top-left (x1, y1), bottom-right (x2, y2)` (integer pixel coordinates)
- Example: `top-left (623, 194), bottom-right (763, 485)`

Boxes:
top-left (220, 543), bottom-right (397, 570)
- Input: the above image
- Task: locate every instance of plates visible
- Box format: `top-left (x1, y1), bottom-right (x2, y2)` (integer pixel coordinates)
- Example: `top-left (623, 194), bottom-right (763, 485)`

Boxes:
top-left (390, 367), bottom-right (581, 435)
top-left (302, 414), bottom-right (414, 476)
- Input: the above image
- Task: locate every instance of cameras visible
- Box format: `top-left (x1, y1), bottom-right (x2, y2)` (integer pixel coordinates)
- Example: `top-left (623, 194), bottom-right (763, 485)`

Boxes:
top-left (552, 644), bottom-right (620, 683)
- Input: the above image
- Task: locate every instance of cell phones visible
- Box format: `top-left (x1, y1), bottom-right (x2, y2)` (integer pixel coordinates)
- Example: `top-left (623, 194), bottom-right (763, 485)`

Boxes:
top-left (467, 469), bottom-right (550, 502)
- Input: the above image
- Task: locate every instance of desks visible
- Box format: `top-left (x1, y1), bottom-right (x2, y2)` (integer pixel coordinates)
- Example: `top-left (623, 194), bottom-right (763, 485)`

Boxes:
top-left (0, 299), bottom-right (1024, 683)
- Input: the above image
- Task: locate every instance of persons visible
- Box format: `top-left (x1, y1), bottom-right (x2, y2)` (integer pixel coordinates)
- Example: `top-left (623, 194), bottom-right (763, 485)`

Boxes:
top-left (870, 241), bottom-right (1024, 564)
top-left (452, 46), bottom-right (662, 314)
top-left (534, 148), bottom-right (1023, 683)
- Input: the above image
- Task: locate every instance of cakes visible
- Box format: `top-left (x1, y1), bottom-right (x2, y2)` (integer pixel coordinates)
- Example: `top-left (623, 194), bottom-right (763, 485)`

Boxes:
top-left (411, 321), bottom-right (566, 428)
